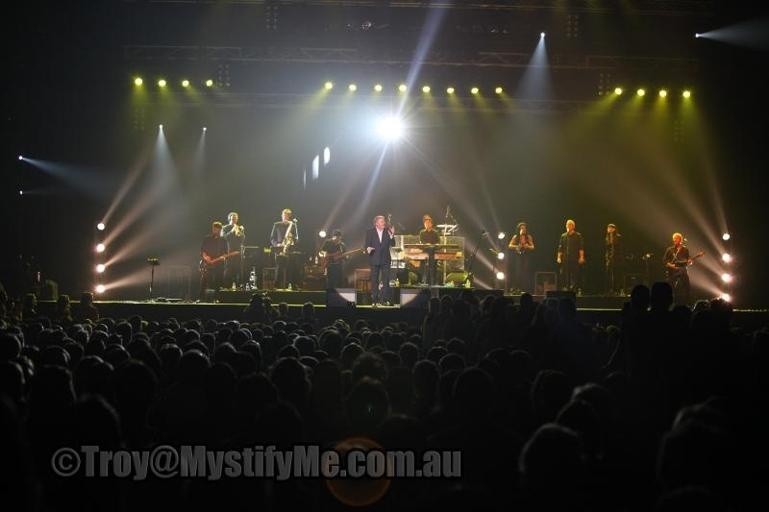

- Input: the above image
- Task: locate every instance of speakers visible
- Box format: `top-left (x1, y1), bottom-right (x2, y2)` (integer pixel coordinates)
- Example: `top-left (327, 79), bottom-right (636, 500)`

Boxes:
top-left (474, 289), bottom-right (504, 304)
top-left (546, 291), bottom-right (576, 311)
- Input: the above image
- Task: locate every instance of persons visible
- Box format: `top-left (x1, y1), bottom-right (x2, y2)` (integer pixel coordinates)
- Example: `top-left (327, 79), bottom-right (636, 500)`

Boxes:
top-left (508, 222), bottom-right (535, 291)
top-left (661, 232), bottom-right (694, 309)
top-left (556, 219), bottom-right (587, 297)
top-left (269, 208), bottom-right (299, 290)
top-left (604, 224), bottom-right (627, 296)
top-left (364, 214), bottom-right (397, 306)
top-left (220, 211), bottom-right (248, 290)
top-left (317, 230), bottom-right (350, 290)
top-left (418, 215), bottom-right (441, 285)
top-left (193, 221), bottom-right (229, 304)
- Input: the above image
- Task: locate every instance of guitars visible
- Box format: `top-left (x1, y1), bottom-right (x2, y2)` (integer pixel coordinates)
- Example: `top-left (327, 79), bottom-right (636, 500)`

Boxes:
top-left (321, 248), bottom-right (363, 269)
top-left (669, 252), bottom-right (704, 275)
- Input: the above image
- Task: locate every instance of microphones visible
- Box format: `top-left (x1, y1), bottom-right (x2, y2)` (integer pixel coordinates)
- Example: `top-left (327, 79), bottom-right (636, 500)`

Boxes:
top-left (384, 224), bottom-right (389, 229)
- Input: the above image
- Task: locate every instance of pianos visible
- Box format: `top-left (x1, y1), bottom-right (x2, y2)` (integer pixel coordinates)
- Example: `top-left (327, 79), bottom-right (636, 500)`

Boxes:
top-left (404, 243), bottom-right (459, 249)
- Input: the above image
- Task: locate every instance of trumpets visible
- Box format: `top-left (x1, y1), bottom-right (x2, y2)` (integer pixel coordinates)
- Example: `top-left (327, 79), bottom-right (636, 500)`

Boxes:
top-left (233, 223), bottom-right (245, 241)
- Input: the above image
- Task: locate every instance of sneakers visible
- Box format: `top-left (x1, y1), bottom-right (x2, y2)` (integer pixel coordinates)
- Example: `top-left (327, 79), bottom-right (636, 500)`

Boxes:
top-left (372, 300), bottom-right (394, 308)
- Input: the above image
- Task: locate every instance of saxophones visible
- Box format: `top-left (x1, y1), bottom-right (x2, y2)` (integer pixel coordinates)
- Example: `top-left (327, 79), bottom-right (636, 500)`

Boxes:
top-left (515, 228), bottom-right (526, 255)
top-left (280, 220), bottom-right (295, 254)
